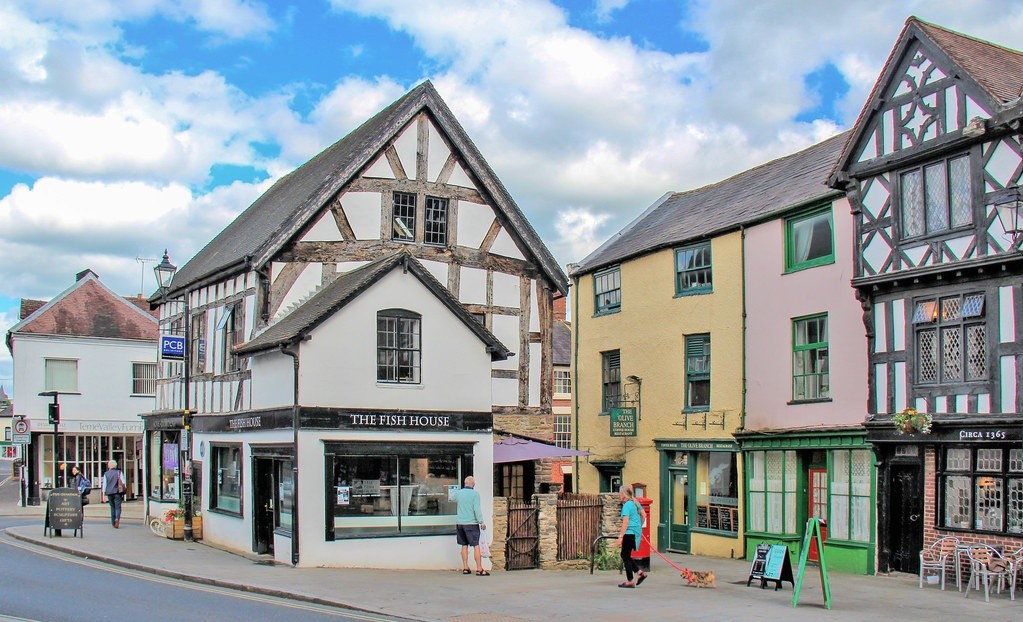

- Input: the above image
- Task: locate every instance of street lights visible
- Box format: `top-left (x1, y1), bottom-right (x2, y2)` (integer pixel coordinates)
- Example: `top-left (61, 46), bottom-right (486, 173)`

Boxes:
top-left (153, 248), bottom-right (194, 544)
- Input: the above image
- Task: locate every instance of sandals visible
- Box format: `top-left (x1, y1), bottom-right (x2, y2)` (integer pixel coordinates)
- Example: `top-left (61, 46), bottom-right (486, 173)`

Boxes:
top-left (463, 568), bottom-right (471, 574)
top-left (477, 569), bottom-right (490, 575)
top-left (618, 582), bottom-right (635, 588)
top-left (636, 571), bottom-right (647, 585)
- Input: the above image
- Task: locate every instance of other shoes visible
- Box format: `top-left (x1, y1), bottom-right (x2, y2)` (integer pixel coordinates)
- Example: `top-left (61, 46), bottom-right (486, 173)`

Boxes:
top-left (112, 518), bottom-right (119, 527)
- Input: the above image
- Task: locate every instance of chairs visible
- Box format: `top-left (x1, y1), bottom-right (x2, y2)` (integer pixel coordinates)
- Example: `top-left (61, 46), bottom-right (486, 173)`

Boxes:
top-left (1004, 544), bottom-right (1023, 597)
top-left (964, 542), bottom-right (1016, 603)
top-left (916, 535), bottom-right (960, 592)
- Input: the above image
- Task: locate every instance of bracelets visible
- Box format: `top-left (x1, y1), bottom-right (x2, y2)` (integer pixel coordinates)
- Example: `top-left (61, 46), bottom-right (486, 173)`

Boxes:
top-left (619, 537), bottom-right (623, 538)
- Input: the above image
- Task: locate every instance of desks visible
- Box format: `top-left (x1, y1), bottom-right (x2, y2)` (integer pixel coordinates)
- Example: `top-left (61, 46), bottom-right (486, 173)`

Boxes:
top-left (956, 543), bottom-right (1005, 592)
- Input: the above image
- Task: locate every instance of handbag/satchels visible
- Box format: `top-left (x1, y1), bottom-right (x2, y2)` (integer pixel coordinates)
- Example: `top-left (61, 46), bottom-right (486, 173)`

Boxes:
top-left (118, 470), bottom-right (127, 494)
top-left (82, 496), bottom-right (89, 505)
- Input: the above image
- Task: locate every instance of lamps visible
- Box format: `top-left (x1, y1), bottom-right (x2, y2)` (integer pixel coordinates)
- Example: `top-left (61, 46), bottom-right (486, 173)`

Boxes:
top-left (625, 375), bottom-right (641, 385)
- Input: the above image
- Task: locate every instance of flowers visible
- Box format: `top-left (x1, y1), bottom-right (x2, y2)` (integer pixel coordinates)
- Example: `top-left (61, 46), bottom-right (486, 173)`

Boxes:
top-left (889, 406), bottom-right (933, 437)
top-left (162, 501), bottom-right (205, 522)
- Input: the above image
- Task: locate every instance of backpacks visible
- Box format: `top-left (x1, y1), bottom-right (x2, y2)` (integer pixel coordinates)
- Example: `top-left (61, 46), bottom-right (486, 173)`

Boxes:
top-left (74, 474), bottom-right (91, 495)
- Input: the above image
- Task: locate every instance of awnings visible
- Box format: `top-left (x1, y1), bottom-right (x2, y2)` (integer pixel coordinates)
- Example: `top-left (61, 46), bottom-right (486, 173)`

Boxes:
top-left (494, 433), bottom-right (599, 462)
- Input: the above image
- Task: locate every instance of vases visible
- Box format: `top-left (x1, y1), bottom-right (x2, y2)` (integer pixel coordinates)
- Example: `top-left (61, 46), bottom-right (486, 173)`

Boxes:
top-left (163, 513), bottom-right (203, 540)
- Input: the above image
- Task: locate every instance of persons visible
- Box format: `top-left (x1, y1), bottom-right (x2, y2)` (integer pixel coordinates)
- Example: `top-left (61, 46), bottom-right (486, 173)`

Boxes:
top-left (101, 460), bottom-right (127, 525)
top-left (618, 484), bottom-right (647, 589)
top-left (455, 475), bottom-right (492, 577)
top-left (69, 465), bottom-right (84, 525)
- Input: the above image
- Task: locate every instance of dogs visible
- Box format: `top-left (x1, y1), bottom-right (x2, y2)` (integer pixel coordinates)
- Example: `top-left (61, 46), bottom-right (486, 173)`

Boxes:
top-left (681, 568), bottom-right (717, 588)
